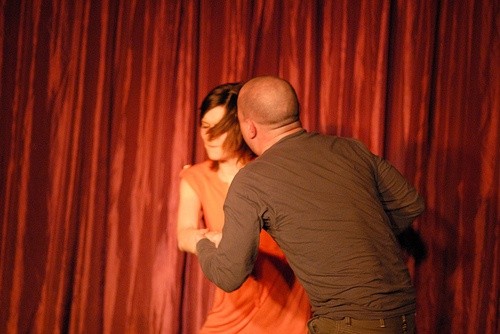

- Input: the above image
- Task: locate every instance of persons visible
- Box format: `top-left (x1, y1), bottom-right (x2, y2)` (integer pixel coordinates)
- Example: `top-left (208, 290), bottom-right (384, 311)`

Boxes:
top-left (176, 80), bottom-right (312, 333)
top-left (194, 76), bottom-right (429, 333)
